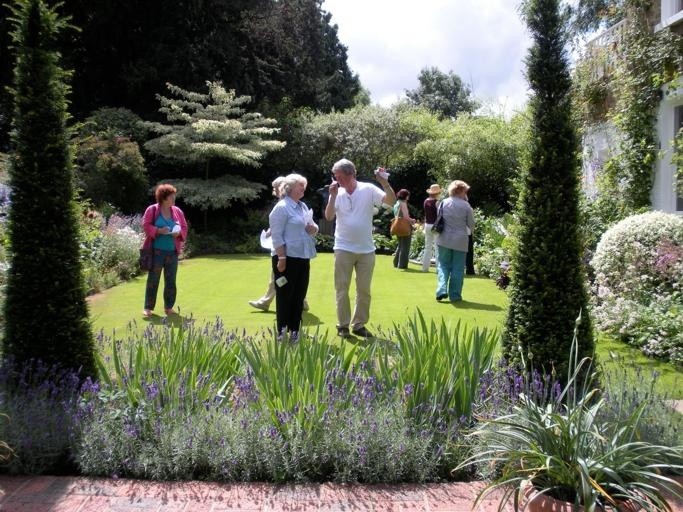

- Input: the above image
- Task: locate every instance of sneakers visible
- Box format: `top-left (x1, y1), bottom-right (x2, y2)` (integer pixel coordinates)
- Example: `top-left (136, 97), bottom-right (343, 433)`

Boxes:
top-left (249, 301), bottom-right (268, 311)
top-left (277, 327), bottom-right (286, 341)
top-left (165, 308), bottom-right (175, 315)
top-left (352, 327), bottom-right (372, 337)
top-left (436, 294), bottom-right (448, 300)
top-left (289, 330), bottom-right (299, 345)
top-left (338, 328), bottom-right (349, 337)
top-left (144, 309), bottom-right (152, 316)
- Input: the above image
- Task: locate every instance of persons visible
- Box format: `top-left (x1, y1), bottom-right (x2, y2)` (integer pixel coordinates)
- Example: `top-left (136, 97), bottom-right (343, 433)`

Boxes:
top-left (139, 183), bottom-right (188, 318)
top-left (247, 175), bottom-right (311, 312)
top-left (323, 157), bottom-right (397, 338)
top-left (389, 188), bottom-right (416, 269)
top-left (268, 172), bottom-right (319, 337)
top-left (421, 183), bottom-right (443, 273)
top-left (435, 179), bottom-right (474, 302)
top-left (465, 225), bottom-right (474, 275)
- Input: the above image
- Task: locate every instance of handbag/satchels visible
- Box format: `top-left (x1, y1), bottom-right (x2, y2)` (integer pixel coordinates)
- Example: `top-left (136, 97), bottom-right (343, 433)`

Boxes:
top-left (431, 216), bottom-right (445, 233)
top-left (390, 217), bottom-right (411, 236)
top-left (139, 249), bottom-right (153, 271)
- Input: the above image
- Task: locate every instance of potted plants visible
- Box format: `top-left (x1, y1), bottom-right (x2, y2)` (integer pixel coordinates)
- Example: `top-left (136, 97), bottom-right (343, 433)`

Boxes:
top-left (450, 310), bottom-right (683, 512)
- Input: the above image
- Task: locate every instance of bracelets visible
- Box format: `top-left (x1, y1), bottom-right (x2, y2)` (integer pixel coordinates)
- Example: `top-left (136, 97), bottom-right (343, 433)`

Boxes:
top-left (277, 256), bottom-right (286, 260)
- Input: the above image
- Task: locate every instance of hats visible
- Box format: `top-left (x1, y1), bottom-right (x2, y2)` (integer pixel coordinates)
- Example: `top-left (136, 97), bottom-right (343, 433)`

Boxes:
top-left (426, 184), bottom-right (444, 194)
